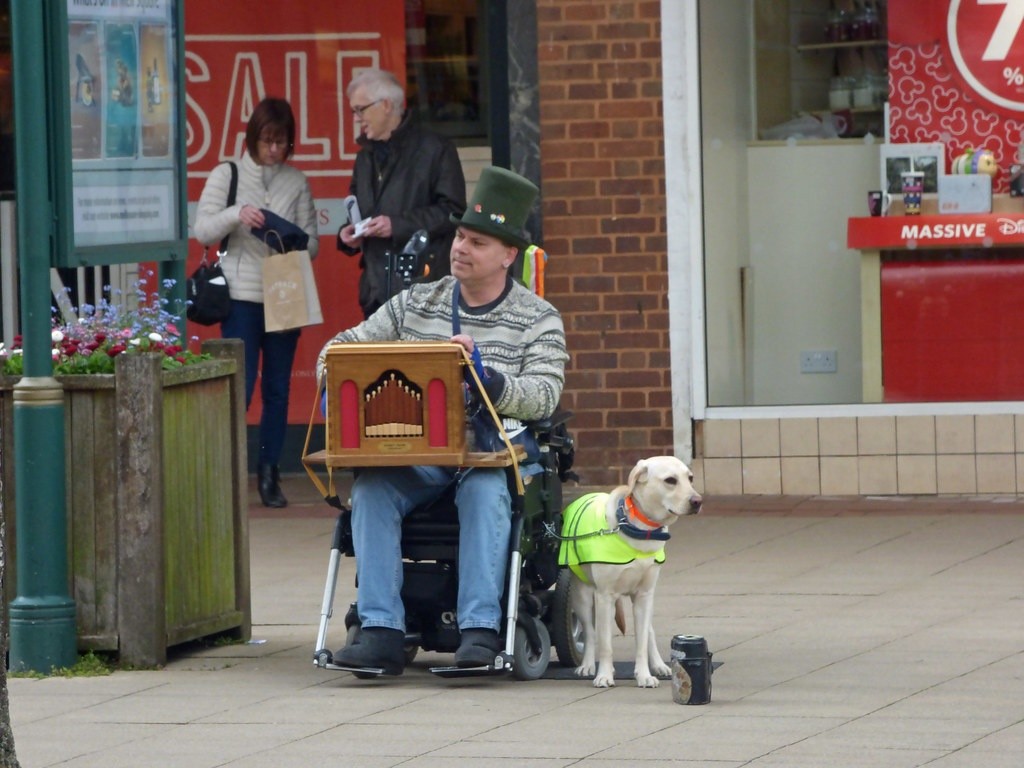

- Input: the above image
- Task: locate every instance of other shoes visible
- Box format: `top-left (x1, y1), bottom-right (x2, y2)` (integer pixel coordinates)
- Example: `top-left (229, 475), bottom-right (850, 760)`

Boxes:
top-left (455, 629), bottom-right (501, 668)
top-left (258, 463), bottom-right (288, 507)
top-left (333, 627), bottom-right (406, 674)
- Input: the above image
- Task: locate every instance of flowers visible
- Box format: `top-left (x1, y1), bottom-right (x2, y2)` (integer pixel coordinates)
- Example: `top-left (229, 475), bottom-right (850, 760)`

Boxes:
top-left (0, 266), bottom-right (215, 374)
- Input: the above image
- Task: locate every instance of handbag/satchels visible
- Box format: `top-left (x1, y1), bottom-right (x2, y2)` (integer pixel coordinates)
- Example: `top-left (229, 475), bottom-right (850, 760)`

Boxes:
top-left (260, 229), bottom-right (324, 333)
top-left (473, 411), bottom-right (541, 464)
top-left (186, 262), bottom-right (231, 327)
top-left (251, 209), bottom-right (310, 254)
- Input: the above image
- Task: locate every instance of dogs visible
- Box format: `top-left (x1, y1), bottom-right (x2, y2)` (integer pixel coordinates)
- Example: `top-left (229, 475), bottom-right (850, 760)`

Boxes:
top-left (569, 455), bottom-right (703, 688)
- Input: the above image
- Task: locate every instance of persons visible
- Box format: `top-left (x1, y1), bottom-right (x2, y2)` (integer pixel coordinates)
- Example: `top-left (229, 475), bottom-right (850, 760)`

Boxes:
top-left (195, 98), bottom-right (321, 506)
top-left (316, 168), bottom-right (570, 676)
top-left (337, 69), bottom-right (467, 321)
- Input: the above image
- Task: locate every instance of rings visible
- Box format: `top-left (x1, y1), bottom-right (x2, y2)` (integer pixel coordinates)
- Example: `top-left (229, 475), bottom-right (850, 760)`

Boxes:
top-left (377, 231), bottom-right (382, 234)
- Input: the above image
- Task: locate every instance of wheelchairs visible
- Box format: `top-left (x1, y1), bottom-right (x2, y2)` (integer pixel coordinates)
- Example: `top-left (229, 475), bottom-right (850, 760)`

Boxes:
top-left (305, 401), bottom-right (588, 682)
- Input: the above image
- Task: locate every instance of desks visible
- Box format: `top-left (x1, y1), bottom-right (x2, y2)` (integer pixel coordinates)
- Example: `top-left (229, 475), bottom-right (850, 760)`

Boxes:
top-left (847, 214), bottom-right (1024, 402)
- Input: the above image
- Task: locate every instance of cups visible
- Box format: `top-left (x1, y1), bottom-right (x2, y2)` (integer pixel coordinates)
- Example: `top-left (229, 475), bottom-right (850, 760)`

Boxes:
top-left (901, 171), bottom-right (925, 215)
top-left (671, 635), bottom-right (711, 705)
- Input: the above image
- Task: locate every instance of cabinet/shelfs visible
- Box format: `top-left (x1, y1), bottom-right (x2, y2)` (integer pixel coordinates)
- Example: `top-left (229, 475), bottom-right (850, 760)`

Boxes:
top-left (795, 39), bottom-right (887, 117)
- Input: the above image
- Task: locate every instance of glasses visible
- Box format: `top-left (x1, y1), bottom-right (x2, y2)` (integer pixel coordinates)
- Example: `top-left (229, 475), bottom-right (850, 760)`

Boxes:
top-left (351, 101), bottom-right (378, 116)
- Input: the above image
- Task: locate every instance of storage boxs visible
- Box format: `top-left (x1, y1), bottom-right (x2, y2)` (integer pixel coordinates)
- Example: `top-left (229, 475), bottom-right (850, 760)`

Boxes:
top-left (936, 174), bottom-right (993, 214)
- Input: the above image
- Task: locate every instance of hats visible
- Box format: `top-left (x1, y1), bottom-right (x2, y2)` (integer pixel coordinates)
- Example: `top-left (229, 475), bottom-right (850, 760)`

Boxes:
top-left (449, 165), bottom-right (540, 249)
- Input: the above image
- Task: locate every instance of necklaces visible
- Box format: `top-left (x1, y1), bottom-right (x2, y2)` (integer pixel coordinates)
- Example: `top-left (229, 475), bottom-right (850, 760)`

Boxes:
top-left (262, 164), bottom-right (280, 205)
